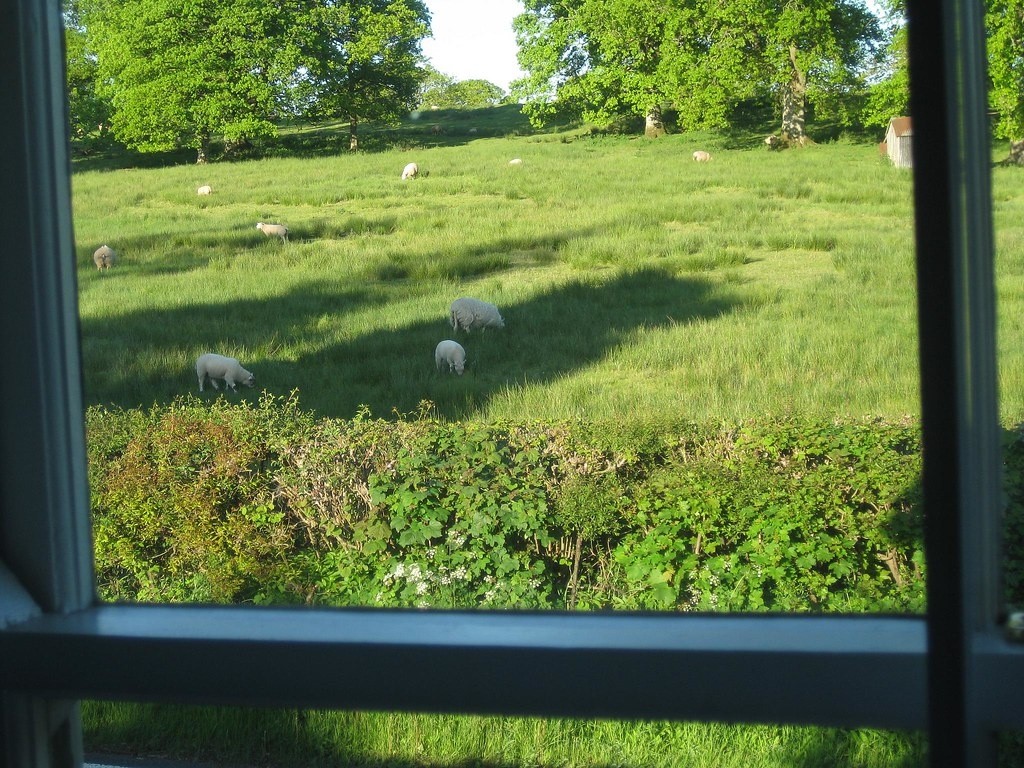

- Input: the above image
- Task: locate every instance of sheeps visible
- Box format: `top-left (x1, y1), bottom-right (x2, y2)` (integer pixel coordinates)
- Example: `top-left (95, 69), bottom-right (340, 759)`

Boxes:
top-left (692, 151), bottom-right (712, 162)
top-left (198, 185), bottom-right (212, 195)
top-left (450, 298), bottom-right (505, 335)
top-left (510, 159), bottom-right (522, 163)
top-left (93, 245), bottom-right (116, 273)
top-left (435, 340), bottom-right (467, 376)
top-left (255, 222), bottom-right (289, 245)
top-left (401, 163), bottom-right (418, 179)
top-left (195, 353), bottom-right (255, 394)
